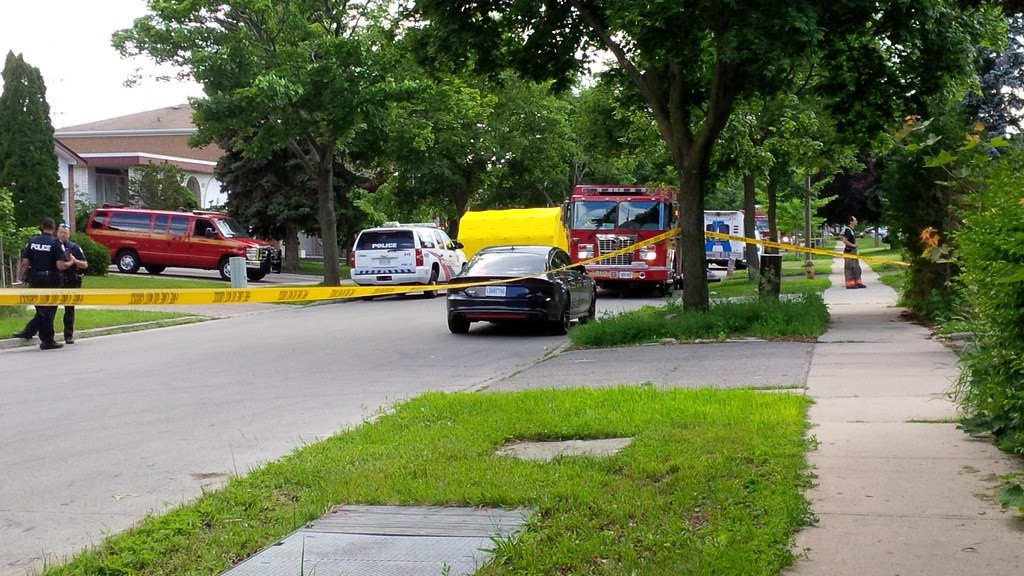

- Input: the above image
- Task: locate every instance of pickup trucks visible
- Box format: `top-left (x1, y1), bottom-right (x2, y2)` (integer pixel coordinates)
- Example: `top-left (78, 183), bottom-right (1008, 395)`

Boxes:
top-left (86, 202), bottom-right (282, 281)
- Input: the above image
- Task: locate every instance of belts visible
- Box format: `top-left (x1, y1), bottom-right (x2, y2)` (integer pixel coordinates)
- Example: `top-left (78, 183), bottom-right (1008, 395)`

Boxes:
top-left (30, 271), bottom-right (50, 276)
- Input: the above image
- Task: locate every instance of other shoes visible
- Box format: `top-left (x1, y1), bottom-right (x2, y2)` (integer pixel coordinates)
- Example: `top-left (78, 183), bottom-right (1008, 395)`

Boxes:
top-left (40, 340), bottom-right (63, 350)
top-left (846, 285), bottom-right (858, 288)
top-left (12, 332), bottom-right (33, 339)
top-left (856, 283), bottom-right (866, 288)
top-left (66, 335), bottom-right (74, 344)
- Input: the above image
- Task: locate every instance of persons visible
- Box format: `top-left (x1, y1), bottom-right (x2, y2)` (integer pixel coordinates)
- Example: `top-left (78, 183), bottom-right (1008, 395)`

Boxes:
top-left (18, 218), bottom-right (74, 350)
top-left (843, 216), bottom-right (866, 289)
top-left (13, 223), bottom-right (88, 343)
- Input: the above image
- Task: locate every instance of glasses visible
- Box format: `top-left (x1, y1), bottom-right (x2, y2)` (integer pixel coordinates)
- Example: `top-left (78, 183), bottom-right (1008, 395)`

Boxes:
top-left (60, 223), bottom-right (70, 228)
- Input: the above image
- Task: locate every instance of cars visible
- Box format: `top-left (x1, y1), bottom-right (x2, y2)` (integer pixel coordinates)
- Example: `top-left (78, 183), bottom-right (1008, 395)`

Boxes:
top-left (447, 243), bottom-right (597, 335)
top-left (860, 226), bottom-right (894, 243)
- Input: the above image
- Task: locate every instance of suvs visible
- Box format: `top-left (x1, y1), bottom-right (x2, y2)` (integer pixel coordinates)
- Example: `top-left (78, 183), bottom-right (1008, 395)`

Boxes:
top-left (349, 221), bottom-right (467, 297)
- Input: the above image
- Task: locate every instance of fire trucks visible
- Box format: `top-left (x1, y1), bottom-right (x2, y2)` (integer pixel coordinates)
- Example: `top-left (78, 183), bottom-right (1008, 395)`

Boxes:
top-left (561, 185), bottom-right (683, 296)
top-left (740, 206), bottom-right (798, 251)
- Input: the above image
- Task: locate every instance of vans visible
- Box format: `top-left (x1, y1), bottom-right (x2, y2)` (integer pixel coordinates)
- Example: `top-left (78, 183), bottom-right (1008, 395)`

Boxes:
top-left (703, 211), bottom-right (764, 268)
